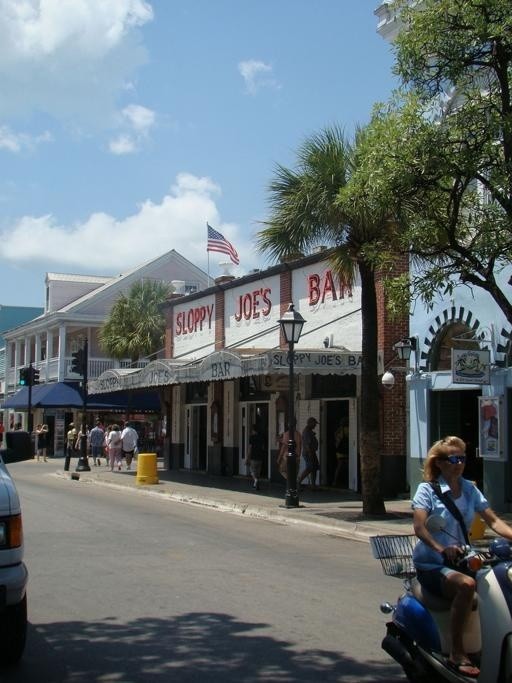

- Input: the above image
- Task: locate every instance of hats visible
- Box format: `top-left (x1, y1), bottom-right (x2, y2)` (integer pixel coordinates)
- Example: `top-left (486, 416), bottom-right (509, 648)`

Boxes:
top-left (69, 423), bottom-right (75, 427)
top-left (308, 417), bottom-right (320, 424)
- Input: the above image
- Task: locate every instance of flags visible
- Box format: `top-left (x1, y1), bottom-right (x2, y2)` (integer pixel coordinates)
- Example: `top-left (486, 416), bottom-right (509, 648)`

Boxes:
top-left (205, 224), bottom-right (240, 264)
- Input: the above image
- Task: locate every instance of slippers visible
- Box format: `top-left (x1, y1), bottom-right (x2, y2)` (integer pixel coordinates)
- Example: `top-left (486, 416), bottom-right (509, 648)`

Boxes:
top-left (447, 655), bottom-right (479, 678)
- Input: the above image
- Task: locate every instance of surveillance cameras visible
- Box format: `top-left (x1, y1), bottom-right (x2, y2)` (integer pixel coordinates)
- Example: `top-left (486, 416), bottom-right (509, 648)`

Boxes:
top-left (382, 371), bottom-right (395, 388)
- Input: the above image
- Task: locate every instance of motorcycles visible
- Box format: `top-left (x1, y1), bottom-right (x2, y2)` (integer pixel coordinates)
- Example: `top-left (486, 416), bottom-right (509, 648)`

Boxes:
top-left (369, 514), bottom-right (511, 681)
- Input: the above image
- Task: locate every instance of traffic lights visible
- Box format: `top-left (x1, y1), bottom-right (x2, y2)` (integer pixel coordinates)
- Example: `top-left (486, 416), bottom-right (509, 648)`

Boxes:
top-left (71, 349), bottom-right (84, 377)
top-left (32, 368), bottom-right (40, 385)
top-left (20, 367), bottom-right (27, 386)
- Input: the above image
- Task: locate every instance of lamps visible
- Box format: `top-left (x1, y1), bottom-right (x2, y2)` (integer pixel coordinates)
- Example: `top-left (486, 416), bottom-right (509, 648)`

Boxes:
top-left (382, 371), bottom-right (395, 390)
top-left (394, 337), bottom-right (415, 360)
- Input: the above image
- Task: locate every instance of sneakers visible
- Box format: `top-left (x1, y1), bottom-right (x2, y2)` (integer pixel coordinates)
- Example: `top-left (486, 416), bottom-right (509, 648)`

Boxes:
top-left (250, 478), bottom-right (259, 489)
top-left (94, 458), bottom-right (121, 472)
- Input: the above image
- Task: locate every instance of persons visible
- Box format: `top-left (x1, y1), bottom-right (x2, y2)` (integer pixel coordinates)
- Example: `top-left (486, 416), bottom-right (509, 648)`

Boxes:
top-left (277, 414), bottom-right (303, 497)
top-left (65, 416), bottom-right (156, 474)
top-left (411, 435), bottom-right (512, 678)
top-left (247, 423), bottom-right (264, 489)
top-left (33, 424), bottom-right (43, 456)
top-left (35, 423), bottom-right (48, 463)
top-left (328, 419), bottom-right (350, 487)
top-left (0, 421), bottom-right (5, 447)
top-left (295, 416), bottom-right (320, 493)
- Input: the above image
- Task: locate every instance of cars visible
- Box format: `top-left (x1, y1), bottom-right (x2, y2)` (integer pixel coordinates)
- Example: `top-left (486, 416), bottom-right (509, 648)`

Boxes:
top-left (1, 448), bottom-right (29, 664)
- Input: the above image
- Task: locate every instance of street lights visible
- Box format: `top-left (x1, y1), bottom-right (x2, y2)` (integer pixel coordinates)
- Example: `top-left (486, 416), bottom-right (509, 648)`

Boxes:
top-left (276, 302), bottom-right (305, 510)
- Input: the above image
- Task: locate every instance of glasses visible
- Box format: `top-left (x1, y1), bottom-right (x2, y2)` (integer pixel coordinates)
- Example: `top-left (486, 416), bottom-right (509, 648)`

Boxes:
top-left (437, 454), bottom-right (466, 465)
